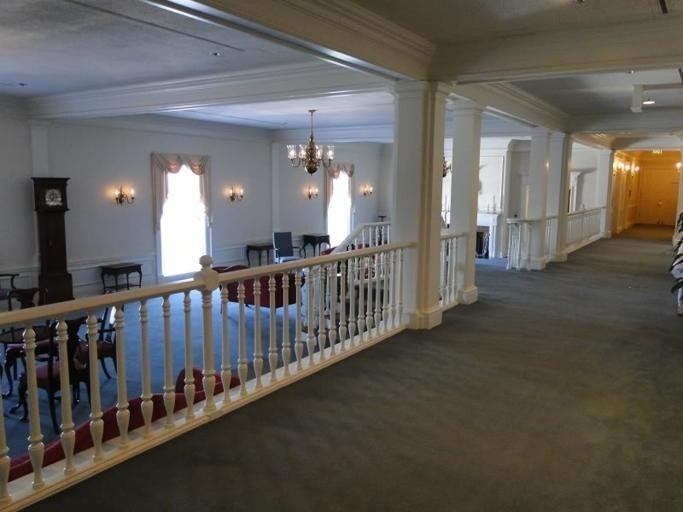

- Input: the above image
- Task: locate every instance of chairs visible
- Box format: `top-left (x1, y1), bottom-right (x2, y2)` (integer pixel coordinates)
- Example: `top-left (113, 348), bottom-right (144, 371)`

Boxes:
top-left (273, 231), bottom-right (301, 263)
top-left (0, 272), bottom-right (126, 436)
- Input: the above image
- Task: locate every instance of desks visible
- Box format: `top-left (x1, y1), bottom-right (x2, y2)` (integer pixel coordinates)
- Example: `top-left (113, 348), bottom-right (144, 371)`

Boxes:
top-left (102, 262), bottom-right (143, 293)
top-left (303, 233), bottom-right (331, 257)
top-left (246, 244), bottom-right (274, 266)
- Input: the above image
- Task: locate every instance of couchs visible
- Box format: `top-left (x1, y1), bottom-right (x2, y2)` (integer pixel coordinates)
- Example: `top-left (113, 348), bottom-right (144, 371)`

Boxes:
top-left (212, 265), bottom-right (305, 316)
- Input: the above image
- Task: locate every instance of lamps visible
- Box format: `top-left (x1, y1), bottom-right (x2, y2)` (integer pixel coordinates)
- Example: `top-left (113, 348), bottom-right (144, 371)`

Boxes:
top-left (286, 110), bottom-right (334, 175)
